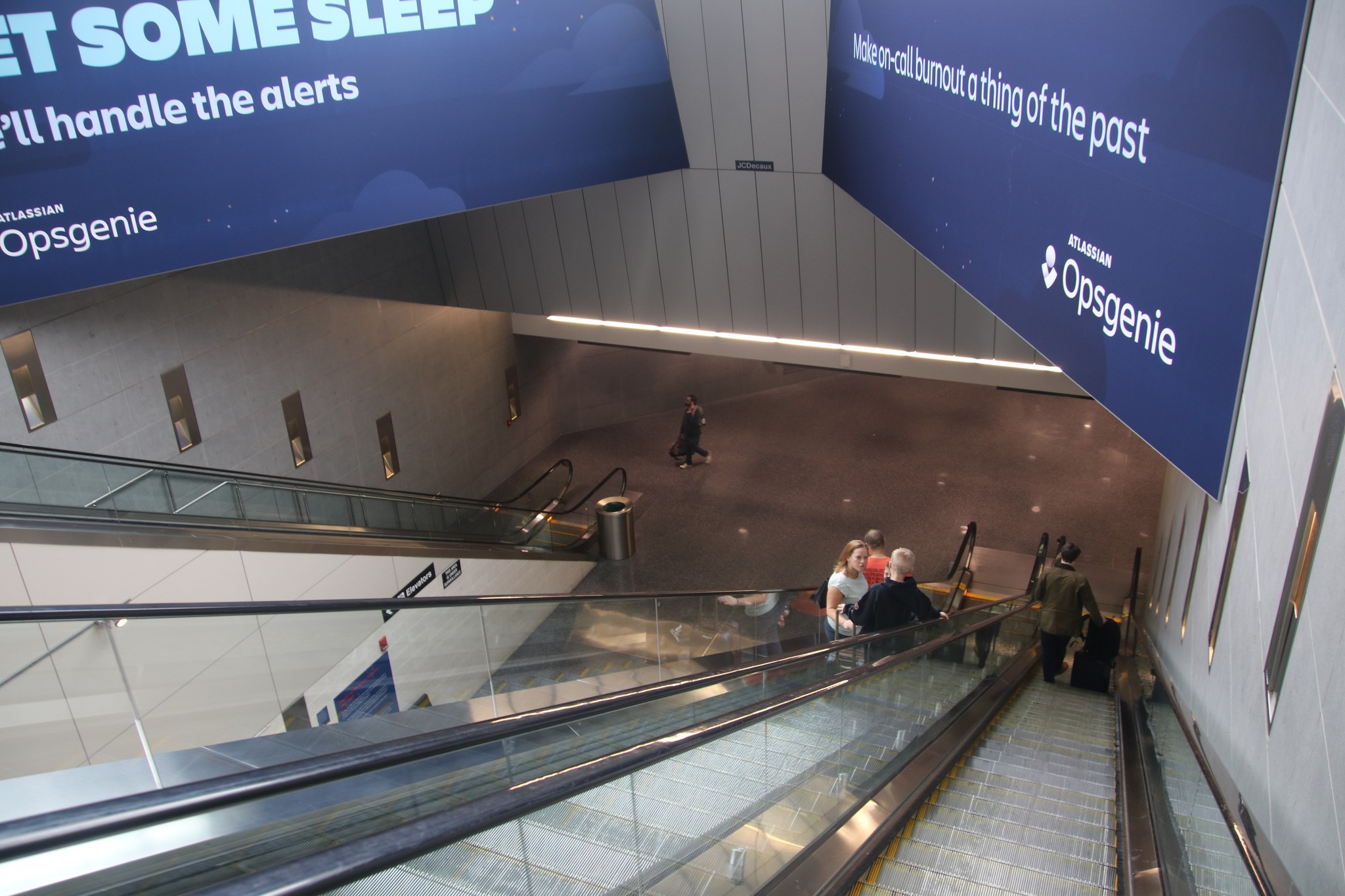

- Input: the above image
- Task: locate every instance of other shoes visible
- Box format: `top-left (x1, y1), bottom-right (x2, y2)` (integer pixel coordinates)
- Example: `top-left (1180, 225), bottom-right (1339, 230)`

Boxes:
top-left (1053, 661), bottom-right (1068, 676)
top-left (679, 463), bottom-right (692, 468)
top-left (705, 451), bottom-right (712, 464)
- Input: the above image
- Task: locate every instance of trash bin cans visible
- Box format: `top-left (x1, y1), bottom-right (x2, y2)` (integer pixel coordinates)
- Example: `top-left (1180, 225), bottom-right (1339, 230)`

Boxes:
top-left (594, 495), bottom-right (635, 559)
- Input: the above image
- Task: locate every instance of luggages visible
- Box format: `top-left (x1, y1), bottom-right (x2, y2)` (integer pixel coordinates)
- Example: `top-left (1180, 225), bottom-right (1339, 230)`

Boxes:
top-left (1070, 647), bottom-right (1114, 693)
top-left (825, 608), bottom-right (865, 677)
top-left (694, 596), bottom-right (742, 666)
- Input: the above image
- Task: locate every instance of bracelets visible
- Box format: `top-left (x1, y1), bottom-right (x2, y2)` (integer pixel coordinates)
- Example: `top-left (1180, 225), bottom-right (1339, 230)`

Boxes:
top-left (736, 598), bottom-right (739, 605)
top-left (842, 620), bottom-right (847, 626)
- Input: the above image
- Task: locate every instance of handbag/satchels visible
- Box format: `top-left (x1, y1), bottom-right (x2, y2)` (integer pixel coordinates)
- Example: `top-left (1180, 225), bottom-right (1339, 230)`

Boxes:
top-left (668, 437), bottom-right (686, 461)
top-left (777, 591), bottom-right (801, 601)
top-left (810, 577), bottom-right (830, 610)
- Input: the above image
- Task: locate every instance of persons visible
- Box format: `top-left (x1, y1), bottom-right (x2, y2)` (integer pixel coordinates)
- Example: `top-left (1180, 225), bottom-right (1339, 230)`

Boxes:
top-left (837, 547), bottom-right (948, 665)
top-left (883, 562), bottom-right (891, 582)
top-left (974, 620), bottom-right (1001, 669)
top-left (718, 590), bottom-right (827, 664)
top-left (862, 529), bottom-right (917, 590)
top-left (679, 395), bottom-right (712, 468)
top-left (824, 540), bottom-right (869, 642)
top-left (1034, 541), bottom-right (1106, 683)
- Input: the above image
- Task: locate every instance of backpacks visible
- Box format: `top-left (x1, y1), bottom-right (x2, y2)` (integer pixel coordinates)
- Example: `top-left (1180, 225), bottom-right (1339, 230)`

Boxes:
top-left (1079, 614), bottom-right (1120, 657)
